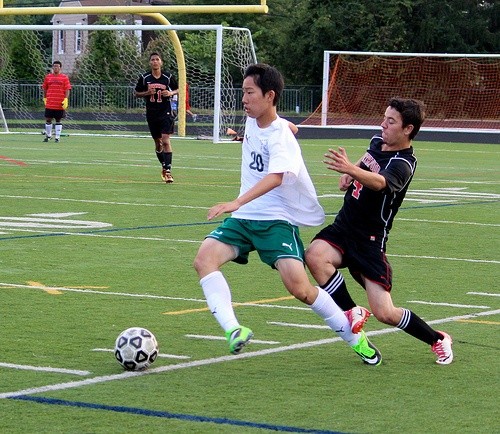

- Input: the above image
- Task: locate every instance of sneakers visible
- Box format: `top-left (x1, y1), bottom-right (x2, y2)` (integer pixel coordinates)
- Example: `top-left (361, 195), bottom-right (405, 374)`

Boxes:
top-left (344, 306), bottom-right (371, 334)
top-left (431, 329), bottom-right (454, 365)
top-left (224, 324), bottom-right (253, 355)
top-left (164, 171), bottom-right (174, 182)
top-left (161, 169), bottom-right (166, 180)
top-left (350, 328), bottom-right (382, 366)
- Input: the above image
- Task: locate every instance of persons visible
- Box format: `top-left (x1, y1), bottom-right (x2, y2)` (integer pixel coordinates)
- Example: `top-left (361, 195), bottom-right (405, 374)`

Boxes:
top-left (305, 96), bottom-right (453, 365)
top-left (169, 78), bottom-right (197, 123)
top-left (42, 61), bottom-right (72, 142)
top-left (194, 63), bottom-right (382, 367)
top-left (134, 54), bottom-right (179, 184)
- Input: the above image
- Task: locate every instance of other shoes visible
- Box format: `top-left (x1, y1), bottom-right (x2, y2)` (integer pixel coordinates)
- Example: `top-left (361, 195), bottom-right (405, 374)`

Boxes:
top-left (55, 138), bottom-right (59, 143)
top-left (192, 114), bottom-right (197, 122)
top-left (43, 136), bottom-right (53, 142)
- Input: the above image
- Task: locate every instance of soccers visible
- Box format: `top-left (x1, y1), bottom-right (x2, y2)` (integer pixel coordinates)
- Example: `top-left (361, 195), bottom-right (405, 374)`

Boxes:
top-left (114, 328), bottom-right (159, 372)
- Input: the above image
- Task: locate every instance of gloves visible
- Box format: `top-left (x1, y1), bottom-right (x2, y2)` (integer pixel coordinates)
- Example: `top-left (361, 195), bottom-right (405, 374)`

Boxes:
top-left (43, 98), bottom-right (47, 105)
top-left (61, 98), bottom-right (68, 109)
